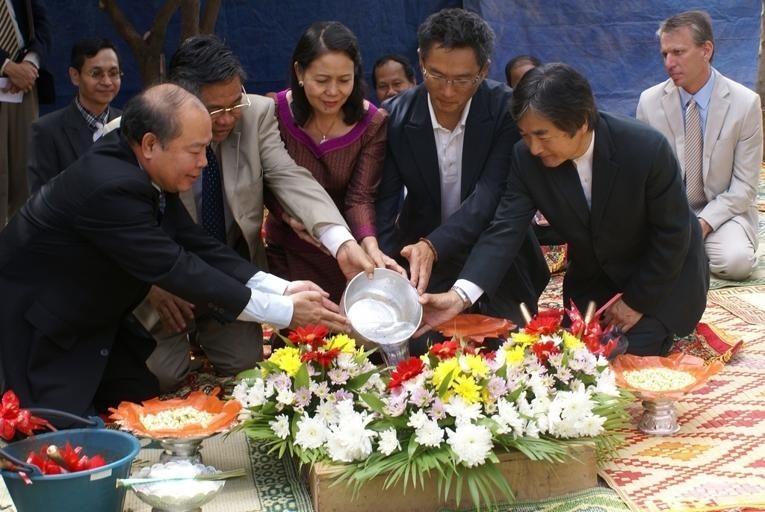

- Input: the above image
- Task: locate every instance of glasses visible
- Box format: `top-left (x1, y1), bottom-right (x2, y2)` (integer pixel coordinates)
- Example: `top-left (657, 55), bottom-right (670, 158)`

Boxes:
top-left (209, 84), bottom-right (252, 120)
top-left (423, 64), bottom-right (480, 85)
top-left (84, 68), bottom-right (124, 78)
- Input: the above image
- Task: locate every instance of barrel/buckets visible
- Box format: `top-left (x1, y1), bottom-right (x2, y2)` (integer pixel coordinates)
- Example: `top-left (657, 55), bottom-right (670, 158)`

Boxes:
top-left (0, 407), bottom-right (141, 512)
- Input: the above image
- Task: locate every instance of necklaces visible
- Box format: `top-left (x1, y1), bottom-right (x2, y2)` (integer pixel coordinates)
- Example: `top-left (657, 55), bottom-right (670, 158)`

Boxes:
top-left (314, 111), bottom-right (341, 144)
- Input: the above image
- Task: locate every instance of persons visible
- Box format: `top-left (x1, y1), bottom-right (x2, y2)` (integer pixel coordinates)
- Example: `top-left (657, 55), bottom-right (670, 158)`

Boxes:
top-left (636, 11), bottom-right (765, 282)
top-left (25, 38), bottom-right (124, 198)
top-left (0, 0), bottom-right (58, 226)
top-left (91, 35), bottom-right (377, 395)
top-left (411, 62), bottom-right (711, 357)
top-left (373, 56), bottom-right (416, 104)
top-left (0, 82), bottom-right (352, 443)
top-left (374, 9), bottom-right (551, 358)
top-left (505, 55), bottom-right (565, 245)
top-left (263, 21), bottom-right (408, 353)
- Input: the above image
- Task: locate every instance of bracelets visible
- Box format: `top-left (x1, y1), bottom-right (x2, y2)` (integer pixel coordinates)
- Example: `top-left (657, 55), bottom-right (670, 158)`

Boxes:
top-left (419, 237), bottom-right (438, 264)
top-left (451, 286), bottom-right (470, 310)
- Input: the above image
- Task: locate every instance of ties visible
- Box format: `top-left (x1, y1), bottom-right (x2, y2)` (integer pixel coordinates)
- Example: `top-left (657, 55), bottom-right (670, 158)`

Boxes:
top-left (202, 142), bottom-right (226, 245)
top-left (685, 99), bottom-right (707, 209)
top-left (0, 0), bottom-right (20, 63)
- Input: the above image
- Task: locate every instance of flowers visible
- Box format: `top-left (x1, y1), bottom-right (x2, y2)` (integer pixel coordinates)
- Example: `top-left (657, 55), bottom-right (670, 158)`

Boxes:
top-left (220, 309), bottom-right (636, 512)
